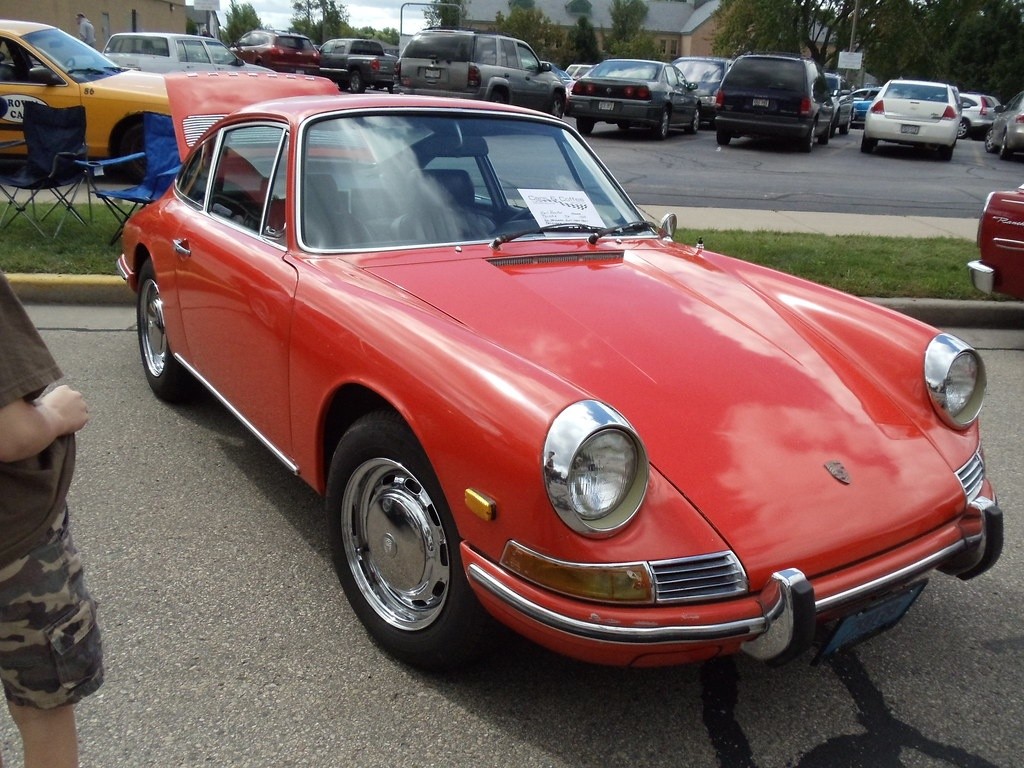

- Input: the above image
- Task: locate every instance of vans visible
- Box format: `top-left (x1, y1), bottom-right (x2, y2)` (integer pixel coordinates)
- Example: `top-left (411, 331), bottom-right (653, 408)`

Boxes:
top-left (671, 56), bottom-right (735, 131)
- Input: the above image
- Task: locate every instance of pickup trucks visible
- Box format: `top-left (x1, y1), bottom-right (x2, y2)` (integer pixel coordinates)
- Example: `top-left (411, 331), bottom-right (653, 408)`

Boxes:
top-left (319, 39), bottom-right (398, 94)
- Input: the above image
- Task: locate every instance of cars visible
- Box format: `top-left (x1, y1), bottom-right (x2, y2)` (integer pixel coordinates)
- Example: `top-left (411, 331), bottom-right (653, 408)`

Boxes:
top-left (860, 78), bottom-right (970, 162)
top-left (565, 58), bottom-right (703, 140)
top-left (986, 91), bottom-right (1024, 158)
top-left (548, 63), bottom-right (597, 89)
top-left (824, 72), bottom-right (855, 138)
top-left (851, 86), bottom-right (883, 121)
top-left (104, 32), bottom-right (277, 73)
top-left (925, 94), bottom-right (1002, 139)
top-left (968, 182), bottom-right (1024, 304)
top-left (0, 19), bottom-right (172, 181)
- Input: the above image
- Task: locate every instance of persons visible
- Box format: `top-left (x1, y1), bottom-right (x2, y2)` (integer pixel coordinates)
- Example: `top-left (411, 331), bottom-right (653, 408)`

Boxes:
top-left (0, 269), bottom-right (107, 768)
top-left (200, 28), bottom-right (215, 38)
top-left (76, 13), bottom-right (97, 48)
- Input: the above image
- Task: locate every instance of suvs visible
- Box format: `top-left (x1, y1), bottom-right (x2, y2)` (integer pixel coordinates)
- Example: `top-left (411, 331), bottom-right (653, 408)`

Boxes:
top-left (230, 28), bottom-right (320, 76)
top-left (393, 27), bottom-right (567, 119)
top-left (714, 51), bottom-right (840, 153)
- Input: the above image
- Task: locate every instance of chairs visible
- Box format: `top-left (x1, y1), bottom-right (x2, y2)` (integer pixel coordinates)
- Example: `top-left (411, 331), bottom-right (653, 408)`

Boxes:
top-left (0, 102), bottom-right (93, 242)
top-left (259, 174), bottom-right (377, 249)
top-left (74, 114), bottom-right (183, 247)
top-left (390, 169), bottom-right (496, 243)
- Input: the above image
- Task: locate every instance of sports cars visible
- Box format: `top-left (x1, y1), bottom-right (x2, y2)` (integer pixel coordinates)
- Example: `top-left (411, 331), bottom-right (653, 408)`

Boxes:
top-left (115, 72), bottom-right (1007, 670)
